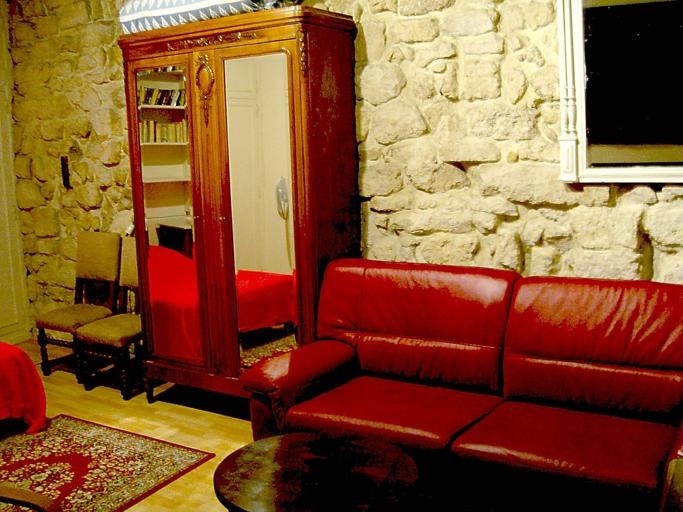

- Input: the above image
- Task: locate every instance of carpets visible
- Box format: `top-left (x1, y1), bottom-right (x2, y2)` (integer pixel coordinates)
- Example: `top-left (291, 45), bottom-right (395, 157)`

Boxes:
top-left (0, 412), bottom-right (215, 512)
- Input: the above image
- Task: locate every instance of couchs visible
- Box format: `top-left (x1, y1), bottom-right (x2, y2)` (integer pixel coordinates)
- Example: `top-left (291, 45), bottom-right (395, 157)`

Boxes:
top-left (238, 256), bottom-right (683, 512)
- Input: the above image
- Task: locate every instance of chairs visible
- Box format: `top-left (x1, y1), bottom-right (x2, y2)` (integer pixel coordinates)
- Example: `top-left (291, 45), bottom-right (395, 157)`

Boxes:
top-left (35, 229), bottom-right (128, 385)
top-left (75, 237), bottom-right (151, 401)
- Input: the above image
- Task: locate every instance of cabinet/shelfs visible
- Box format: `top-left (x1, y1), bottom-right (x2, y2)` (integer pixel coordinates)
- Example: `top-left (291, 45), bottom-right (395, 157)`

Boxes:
top-left (134, 66), bottom-right (194, 250)
top-left (116, 5), bottom-right (359, 406)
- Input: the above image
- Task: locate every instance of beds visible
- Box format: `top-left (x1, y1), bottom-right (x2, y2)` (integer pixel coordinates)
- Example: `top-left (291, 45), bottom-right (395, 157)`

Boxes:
top-left (147, 243), bottom-right (298, 364)
top-left (0, 339), bottom-right (46, 435)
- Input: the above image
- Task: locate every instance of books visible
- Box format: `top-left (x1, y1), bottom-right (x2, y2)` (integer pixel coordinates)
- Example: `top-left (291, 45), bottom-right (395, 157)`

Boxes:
top-left (132, 66), bottom-right (189, 145)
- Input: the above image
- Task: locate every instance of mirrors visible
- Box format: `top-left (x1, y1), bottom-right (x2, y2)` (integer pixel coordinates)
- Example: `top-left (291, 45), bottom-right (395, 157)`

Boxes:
top-left (224, 52), bottom-right (297, 377)
top-left (133, 65), bottom-right (208, 367)
